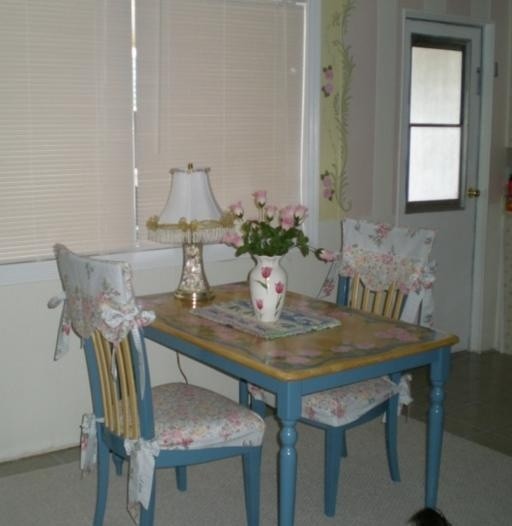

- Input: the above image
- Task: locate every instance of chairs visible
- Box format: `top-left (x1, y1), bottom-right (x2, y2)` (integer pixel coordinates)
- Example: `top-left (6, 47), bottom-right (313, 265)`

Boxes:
top-left (248, 220), bottom-right (438, 516)
top-left (53, 243), bottom-right (267, 526)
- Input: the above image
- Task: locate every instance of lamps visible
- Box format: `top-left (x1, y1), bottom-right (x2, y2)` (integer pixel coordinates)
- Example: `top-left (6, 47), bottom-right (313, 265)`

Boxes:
top-left (146, 165), bottom-right (236, 304)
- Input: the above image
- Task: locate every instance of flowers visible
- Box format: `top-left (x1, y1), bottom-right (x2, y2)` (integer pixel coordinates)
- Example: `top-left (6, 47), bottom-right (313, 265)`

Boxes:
top-left (219, 189), bottom-right (336, 264)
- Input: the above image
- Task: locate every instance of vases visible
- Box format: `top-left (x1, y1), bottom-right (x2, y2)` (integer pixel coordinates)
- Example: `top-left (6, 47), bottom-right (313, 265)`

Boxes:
top-left (246, 254), bottom-right (289, 323)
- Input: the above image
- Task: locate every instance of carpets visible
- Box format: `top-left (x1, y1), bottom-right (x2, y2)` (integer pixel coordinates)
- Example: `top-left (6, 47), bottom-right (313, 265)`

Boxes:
top-left (1, 414), bottom-right (511, 526)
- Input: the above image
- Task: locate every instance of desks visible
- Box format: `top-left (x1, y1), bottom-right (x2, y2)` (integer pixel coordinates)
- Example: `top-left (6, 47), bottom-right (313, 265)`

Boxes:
top-left (123, 279), bottom-right (459, 526)
